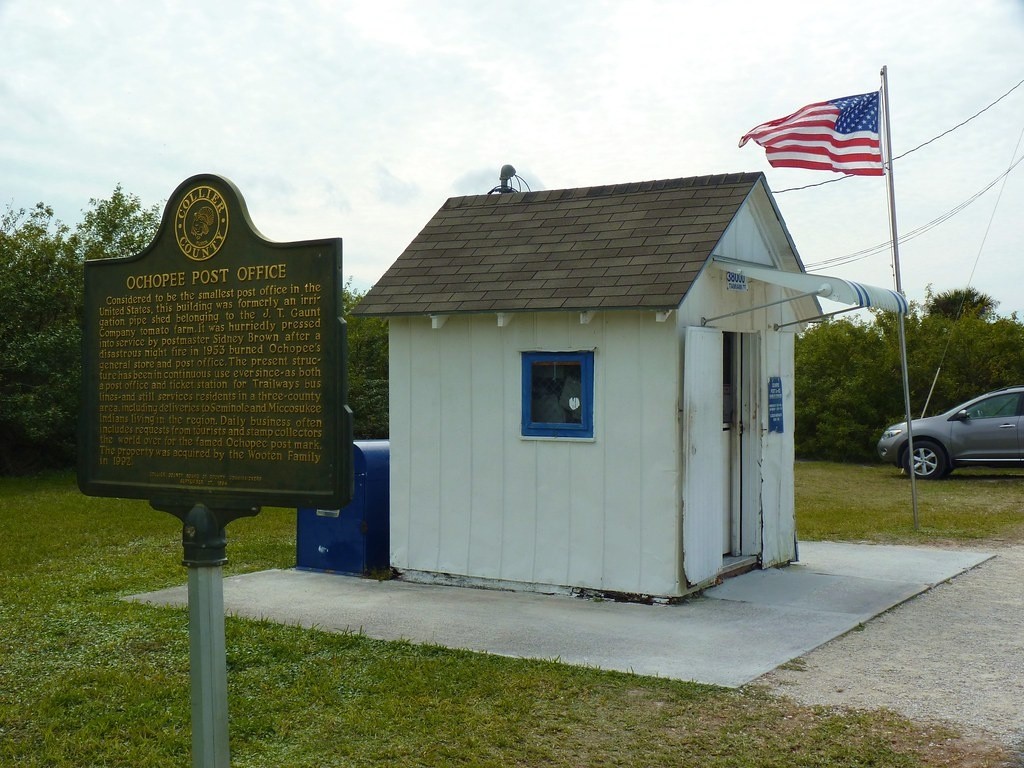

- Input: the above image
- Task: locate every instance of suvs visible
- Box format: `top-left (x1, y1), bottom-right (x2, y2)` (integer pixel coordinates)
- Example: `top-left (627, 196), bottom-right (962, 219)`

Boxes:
top-left (876, 384), bottom-right (1024, 480)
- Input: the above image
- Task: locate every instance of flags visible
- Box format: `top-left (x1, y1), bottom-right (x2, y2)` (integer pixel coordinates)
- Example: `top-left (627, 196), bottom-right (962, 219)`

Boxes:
top-left (739, 89), bottom-right (889, 175)
